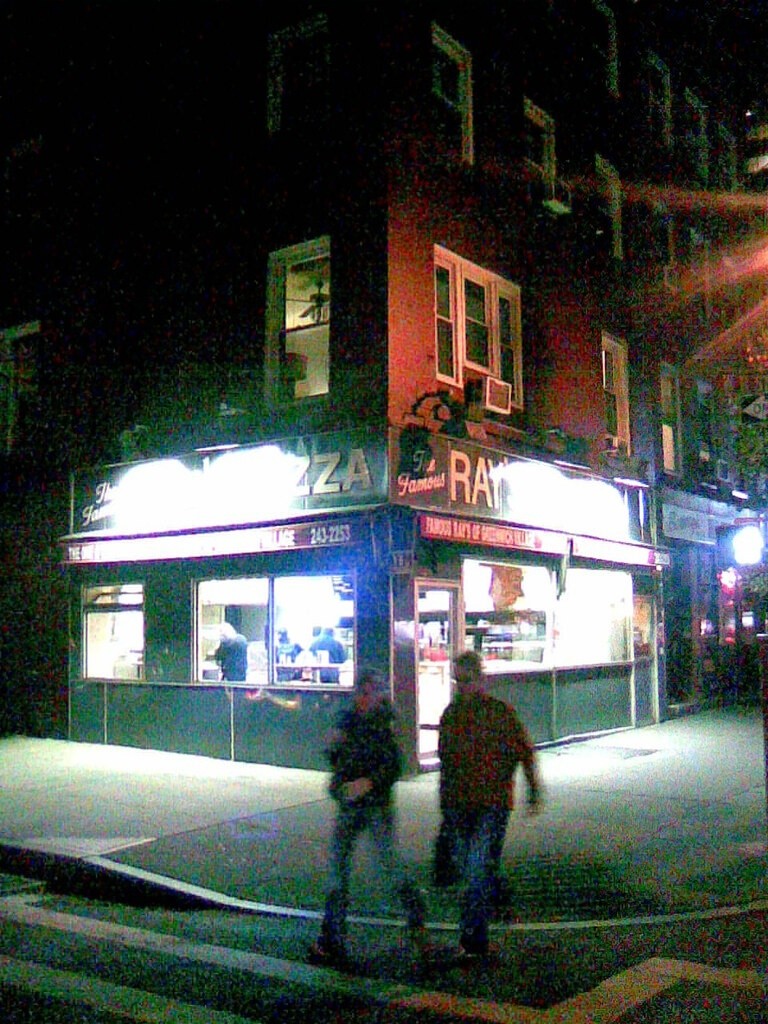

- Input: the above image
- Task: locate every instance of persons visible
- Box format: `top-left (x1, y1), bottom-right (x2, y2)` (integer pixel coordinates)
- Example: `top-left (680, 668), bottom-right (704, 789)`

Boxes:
top-left (276, 623), bottom-right (348, 685)
top-left (303, 666), bottom-right (442, 973)
top-left (215, 605), bottom-right (248, 682)
top-left (437, 651), bottom-right (543, 965)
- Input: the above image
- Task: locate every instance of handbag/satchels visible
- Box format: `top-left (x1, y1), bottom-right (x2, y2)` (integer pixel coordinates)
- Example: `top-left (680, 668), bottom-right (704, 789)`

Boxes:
top-left (429, 821), bottom-right (462, 888)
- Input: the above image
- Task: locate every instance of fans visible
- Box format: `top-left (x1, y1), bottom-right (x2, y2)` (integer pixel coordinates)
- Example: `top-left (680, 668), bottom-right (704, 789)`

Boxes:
top-left (287, 279), bottom-right (330, 325)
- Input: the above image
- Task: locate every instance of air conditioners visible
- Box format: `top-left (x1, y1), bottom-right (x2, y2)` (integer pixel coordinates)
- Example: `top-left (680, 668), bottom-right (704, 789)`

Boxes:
top-left (714, 460), bottom-right (731, 483)
top-left (475, 376), bottom-right (511, 414)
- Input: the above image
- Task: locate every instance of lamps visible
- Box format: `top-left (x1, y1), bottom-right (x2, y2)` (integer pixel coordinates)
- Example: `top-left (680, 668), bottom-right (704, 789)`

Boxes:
top-left (544, 429), bottom-right (589, 470)
top-left (598, 448), bottom-right (652, 489)
top-left (414, 391), bottom-right (486, 442)
